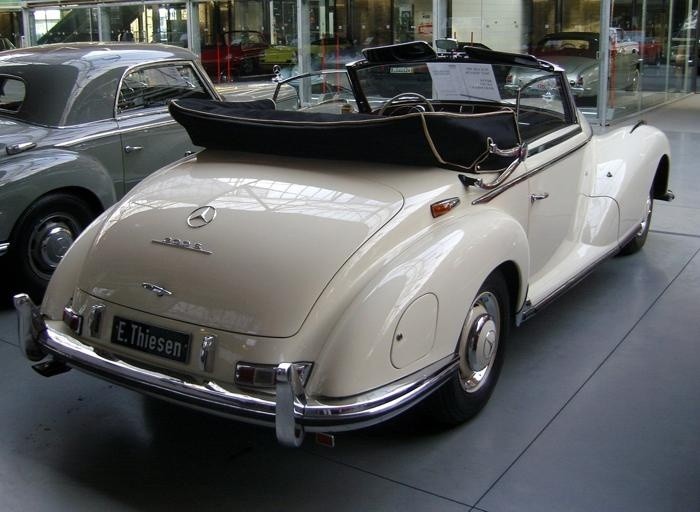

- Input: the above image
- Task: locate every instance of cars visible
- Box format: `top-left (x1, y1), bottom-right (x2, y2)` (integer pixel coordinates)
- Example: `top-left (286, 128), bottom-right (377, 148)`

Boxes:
top-left (506, 32), bottom-right (642, 100)
top-left (259, 37), bottom-right (355, 79)
top-left (11, 40), bottom-right (676, 449)
top-left (278, 44), bottom-right (377, 93)
top-left (671, 27), bottom-right (700, 62)
top-left (609, 28), bottom-right (663, 66)
top-left (0, 40), bottom-right (299, 293)
top-left (201, 30), bottom-right (270, 90)
top-left (367, 37), bottom-right (513, 99)
